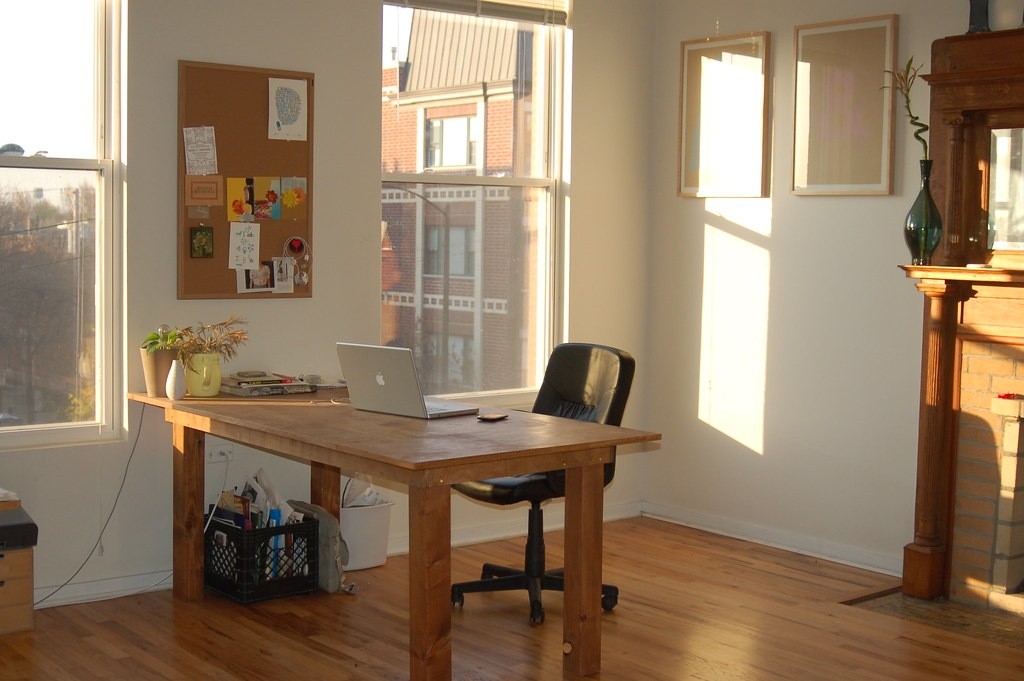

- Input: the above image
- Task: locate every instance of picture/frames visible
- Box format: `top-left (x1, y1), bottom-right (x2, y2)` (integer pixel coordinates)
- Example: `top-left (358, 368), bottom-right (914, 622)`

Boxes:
top-left (788, 13), bottom-right (900, 196)
top-left (675, 30), bottom-right (770, 199)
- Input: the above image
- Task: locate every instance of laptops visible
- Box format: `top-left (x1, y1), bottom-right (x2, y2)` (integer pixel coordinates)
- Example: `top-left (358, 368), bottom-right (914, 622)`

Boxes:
top-left (335, 342), bottom-right (479, 419)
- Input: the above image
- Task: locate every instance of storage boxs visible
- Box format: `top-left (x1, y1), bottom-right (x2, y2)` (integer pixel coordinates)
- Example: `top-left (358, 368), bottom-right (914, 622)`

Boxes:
top-left (204, 513), bottom-right (319, 607)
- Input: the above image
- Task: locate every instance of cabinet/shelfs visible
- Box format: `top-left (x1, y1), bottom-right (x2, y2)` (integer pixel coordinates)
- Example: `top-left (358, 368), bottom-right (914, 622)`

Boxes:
top-left (0, 498), bottom-right (38, 635)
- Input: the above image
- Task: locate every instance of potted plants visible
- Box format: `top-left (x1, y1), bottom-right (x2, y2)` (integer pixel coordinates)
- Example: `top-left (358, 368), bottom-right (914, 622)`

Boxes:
top-left (139, 331), bottom-right (186, 397)
top-left (170, 312), bottom-right (249, 397)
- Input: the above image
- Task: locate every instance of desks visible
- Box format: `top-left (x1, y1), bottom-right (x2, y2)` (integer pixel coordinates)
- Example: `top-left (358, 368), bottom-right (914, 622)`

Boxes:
top-left (128, 387), bottom-right (665, 681)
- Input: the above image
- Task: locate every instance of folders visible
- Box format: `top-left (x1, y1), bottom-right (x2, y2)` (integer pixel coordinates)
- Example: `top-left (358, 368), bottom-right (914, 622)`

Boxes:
top-left (268, 509), bottom-right (281, 577)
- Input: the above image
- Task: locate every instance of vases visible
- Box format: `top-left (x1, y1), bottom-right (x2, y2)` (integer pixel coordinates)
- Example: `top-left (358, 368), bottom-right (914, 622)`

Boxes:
top-left (166, 359), bottom-right (188, 400)
top-left (904, 160), bottom-right (943, 266)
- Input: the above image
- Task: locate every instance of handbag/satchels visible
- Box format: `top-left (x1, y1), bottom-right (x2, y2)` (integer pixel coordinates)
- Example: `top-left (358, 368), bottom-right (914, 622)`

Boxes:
top-left (286, 499), bottom-right (349, 594)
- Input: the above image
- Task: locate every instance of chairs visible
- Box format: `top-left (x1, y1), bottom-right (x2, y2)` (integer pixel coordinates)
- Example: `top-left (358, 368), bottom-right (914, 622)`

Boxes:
top-left (451, 342), bottom-right (636, 627)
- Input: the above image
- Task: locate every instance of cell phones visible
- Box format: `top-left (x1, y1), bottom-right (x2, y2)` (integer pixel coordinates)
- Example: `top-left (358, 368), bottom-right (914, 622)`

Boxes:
top-left (477, 413), bottom-right (508, 422)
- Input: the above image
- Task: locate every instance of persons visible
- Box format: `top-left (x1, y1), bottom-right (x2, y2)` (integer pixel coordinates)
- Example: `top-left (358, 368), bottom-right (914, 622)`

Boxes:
top-left (278, 261), bottom-right (285, 281)
top-left (248, 263), bottom-right (271, 289)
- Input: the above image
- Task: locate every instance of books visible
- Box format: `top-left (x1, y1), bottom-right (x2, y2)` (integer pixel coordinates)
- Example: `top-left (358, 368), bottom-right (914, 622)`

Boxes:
top-left (209, 468), bottom-right (307, 579)
top-left (220, 371), bottom-right (318, 397)
